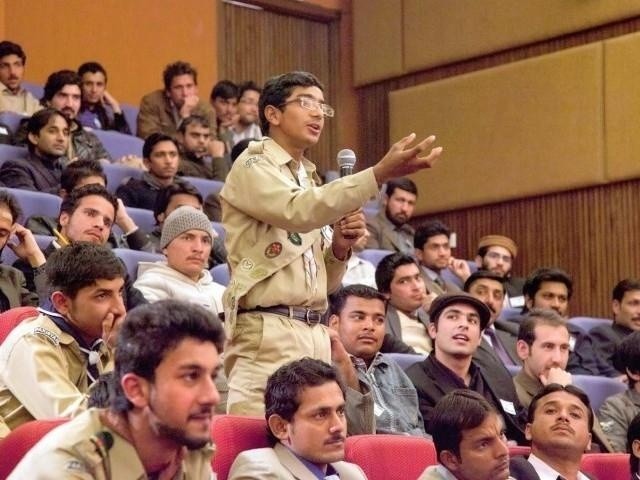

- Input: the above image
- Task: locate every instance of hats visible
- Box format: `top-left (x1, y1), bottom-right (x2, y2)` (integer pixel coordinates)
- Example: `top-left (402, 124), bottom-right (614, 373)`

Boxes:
top-left (428, 292), bottom-right (491, 331)
top-left (464, 270), bottom-right (511, 289)
top-left (160, 204), bottom-right (215, 255)
top-left (476, 235), bottom-right (518, 260)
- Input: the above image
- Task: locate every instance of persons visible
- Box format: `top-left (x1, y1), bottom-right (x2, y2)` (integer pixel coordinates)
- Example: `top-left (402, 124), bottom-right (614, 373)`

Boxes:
top-left (0, 41), bottom-right (640, 479)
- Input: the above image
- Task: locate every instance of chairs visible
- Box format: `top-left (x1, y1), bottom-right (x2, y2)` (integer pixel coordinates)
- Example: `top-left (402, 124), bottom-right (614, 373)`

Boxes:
top-left (0, 82), bottom-right (632, 480)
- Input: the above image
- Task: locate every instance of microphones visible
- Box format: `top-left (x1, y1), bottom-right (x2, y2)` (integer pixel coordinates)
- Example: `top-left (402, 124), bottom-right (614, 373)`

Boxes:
top-left (337, 149), bottom-right (357, 240)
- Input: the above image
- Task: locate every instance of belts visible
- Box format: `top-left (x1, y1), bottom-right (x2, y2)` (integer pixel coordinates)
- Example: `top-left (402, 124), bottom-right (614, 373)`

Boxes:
top-left (238, 304), bottom-right (331, 326)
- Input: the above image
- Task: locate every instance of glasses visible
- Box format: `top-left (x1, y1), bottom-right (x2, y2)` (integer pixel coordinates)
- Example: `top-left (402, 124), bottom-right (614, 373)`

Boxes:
top-left (277, 96), bottom-right (336, 118)
